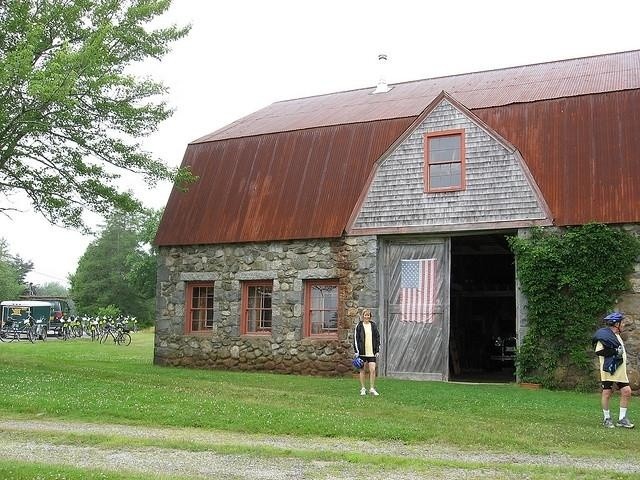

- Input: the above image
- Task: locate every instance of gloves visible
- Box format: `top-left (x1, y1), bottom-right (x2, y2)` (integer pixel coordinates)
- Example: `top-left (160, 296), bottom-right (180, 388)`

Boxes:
top-left (616, 346), bottom-right (626, 360)
top-left (374, 353), bottom-right (380, 359)
top-left (353, 353), bottom-right (359, 358)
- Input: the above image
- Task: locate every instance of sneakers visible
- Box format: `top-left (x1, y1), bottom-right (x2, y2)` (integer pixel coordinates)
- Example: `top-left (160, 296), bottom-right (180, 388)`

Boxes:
top-left (360, 388), bottom-right (379, 395)
top-left (603, 417), bottom-right (634, 428)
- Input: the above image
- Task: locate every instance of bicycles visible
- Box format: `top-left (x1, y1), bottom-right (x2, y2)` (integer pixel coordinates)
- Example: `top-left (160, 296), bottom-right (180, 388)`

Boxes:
top-left (1, 314), bottom-right (131, 345)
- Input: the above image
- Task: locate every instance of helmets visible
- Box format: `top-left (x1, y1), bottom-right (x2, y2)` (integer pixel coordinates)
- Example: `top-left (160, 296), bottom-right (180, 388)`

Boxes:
top-left (352, 358), bottom-right (364, 369)
top-left (604, 312), bottom-right (625, 322)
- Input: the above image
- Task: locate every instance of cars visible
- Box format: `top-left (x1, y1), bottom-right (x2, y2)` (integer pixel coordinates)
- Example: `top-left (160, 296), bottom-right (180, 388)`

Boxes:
top-left (485, 307), bottom-right (516, 370)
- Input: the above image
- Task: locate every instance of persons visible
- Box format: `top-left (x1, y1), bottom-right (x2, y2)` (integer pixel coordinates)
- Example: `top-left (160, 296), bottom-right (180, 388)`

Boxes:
top-left (595, 313), bottom-right (634, 428)
top-left (353, 309), bottom-right (380, 396)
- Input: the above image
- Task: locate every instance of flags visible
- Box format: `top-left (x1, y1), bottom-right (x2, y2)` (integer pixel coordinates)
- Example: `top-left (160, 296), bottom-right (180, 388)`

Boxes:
top-left (400, 259), bottom-right (435, 324)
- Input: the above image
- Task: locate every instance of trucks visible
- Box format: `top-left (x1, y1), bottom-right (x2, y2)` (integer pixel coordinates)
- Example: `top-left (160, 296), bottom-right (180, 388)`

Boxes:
top-left (0, 300), bottom-right (70, 339)
